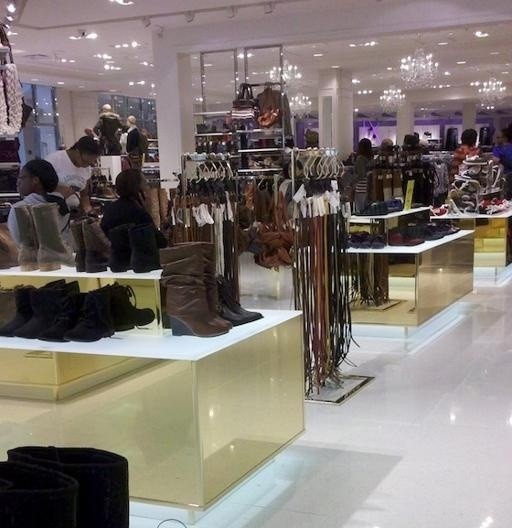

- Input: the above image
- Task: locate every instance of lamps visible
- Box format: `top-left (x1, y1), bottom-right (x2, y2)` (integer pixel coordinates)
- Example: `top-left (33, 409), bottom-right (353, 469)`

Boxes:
top-left (381, 85), bottom-right (404, 114)
top-left (471, 77), bottom-right (508, 111)
top-left (399, 49), bottom-right (440, 89)
top-left (269, 60), bottom-right (302, 86)
top-left (289, 90), bottom-right (311, 113)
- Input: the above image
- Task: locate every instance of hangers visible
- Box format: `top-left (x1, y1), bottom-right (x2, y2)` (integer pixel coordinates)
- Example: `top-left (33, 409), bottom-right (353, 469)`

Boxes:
top-left (180, 152), bottom-right (233, 181)
top-left (400, 150), bottom-right (446, 161)
top-left (286, 147), bottom-right (341, 181)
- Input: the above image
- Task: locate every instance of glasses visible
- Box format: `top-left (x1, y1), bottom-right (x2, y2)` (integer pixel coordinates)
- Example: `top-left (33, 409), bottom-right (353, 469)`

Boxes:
top-left (78, 148), bottom-right (95, 168)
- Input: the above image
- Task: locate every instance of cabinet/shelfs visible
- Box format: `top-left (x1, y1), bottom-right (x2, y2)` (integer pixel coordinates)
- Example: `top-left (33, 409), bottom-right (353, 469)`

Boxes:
top-left (140, 137), bottom-right (161, 178)
top-left (344, 205), bottom-right (475, 326)
top-left (429, 152), bottom-right (511, 266)
top-left (0, 267), bottom-right (306, 526)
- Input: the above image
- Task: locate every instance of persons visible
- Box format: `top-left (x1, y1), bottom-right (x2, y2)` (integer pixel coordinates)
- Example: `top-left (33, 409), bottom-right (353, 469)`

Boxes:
top-left (347, 138), bottom-right (374, 211)
top-left (493, 128), bottom-right (512, 179)
top-left (93, 104), bottom-right (129, 155)
top-left (100, 169), bottom-right (170, 248)
top-left (449, 129), bottom-right (483, 185)
top-left (6, 159), bottom-right (74, 253)
top-left (120, 125), bottom-right (130, 154)
top-left (43, 136), bottom-right (99, 214)
top-left (127, 116), bottom-right (144, 175)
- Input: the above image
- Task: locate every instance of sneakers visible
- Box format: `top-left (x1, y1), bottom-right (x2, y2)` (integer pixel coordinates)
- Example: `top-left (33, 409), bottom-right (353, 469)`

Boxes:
top-left (430, 152), bottom-right (512, 215)
top-left (372, 234), bottom-right (385, 249)
top-left (352, 230), bottom-right (362, 248)
top-left (362, 231), bottom-right (372, 249)
top-left (387, 199), bottom-right (404, 212)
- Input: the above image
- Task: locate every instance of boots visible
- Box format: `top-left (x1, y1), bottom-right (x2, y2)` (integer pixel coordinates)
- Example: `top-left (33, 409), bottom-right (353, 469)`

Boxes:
top-left (0, 461), bottom-right (78, 528)
top-left (199, 243), bottom-right (234, 331)
top-left (63, 289), bottom-right (116, 342)
top-left (0, 287), bottom-right (31, 337)
top-left (81, 218), bottom-right (112, 273)
top-left (70, 220), bottom-right (85, 272)
top-left (32, 202), bottom-right (75, 272)
top-left (8, 446), bottom-right (130, 527)
top-left (110, 223), bottom-right (134, 273)
top-left (37, 289), bottom-right (82, 340)
top-left (103, 280), bottom-right (155, 332)
top-left (159, 242), bottom-right (229, 338)
top-left (13, 205), bottom-right (38, 271)
top-left (14, 286), bottom-right (55, 339)
top-left (129, 222), bottom-right (159, 273)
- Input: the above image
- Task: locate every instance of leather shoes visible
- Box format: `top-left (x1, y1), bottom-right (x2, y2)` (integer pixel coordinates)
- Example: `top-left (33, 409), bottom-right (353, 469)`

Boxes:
top-left (403, 231), bottom-right (423, 245)
top-left (388, 228), bottom-right (403, 246)
top-left (418, 223), bottom-right (460, 239)
top-left (218, 275), bottom-right (263, 327)
top-left (356, 201), bottom-right (387, 215)
top-left (218, 298), bottom-right (251, 326)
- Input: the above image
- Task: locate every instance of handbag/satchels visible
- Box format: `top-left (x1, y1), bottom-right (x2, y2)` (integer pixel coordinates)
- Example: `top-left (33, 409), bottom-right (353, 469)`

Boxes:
top-left (231, 82), bottom-right (261, 121)
top-left (258, 84), bottom-right (281, 126)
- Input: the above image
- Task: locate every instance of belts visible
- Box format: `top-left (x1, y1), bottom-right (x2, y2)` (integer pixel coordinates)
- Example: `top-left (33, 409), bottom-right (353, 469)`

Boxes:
top-left (186, 178), bottom-right (235, 279)
top-left (171, 183), bottom-right (183, 242)
top-left (352, 252), bottom-right (389, 306)
top-left (353, 338), bottom-right (362, 350)
top-left (294, 181), bottom-right (352, 397)
